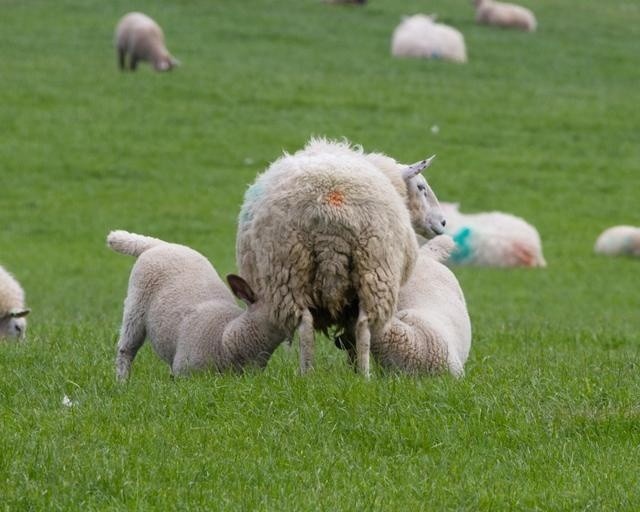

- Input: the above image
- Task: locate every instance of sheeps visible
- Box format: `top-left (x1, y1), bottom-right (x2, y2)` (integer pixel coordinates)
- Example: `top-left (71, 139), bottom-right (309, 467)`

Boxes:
top-left (390, 12), bottom-right (468, 65)
top-left (116, 11), bottom-right (181, 71)
top-left (236, 135), bottom-right (547, 379)
top-left (0, 265), bottom-right (31, 343)
top-left (475, 0), bottom-right (537, 33)
top-left (595, 225), bottom-right (640, 257)
top-left (108, 230), bottom-right (300, 396)
